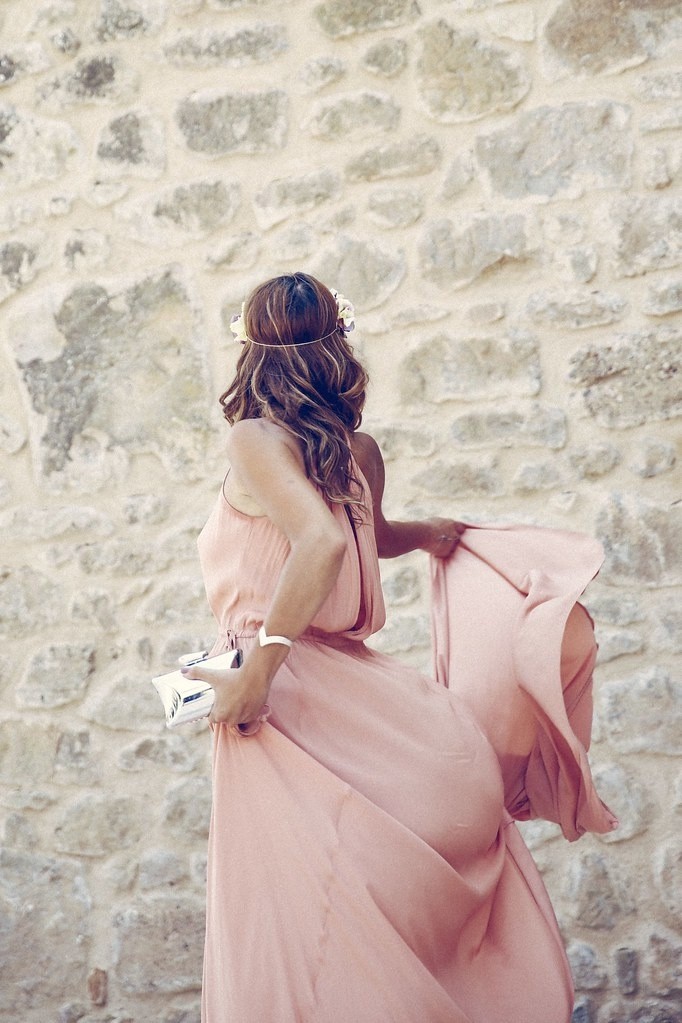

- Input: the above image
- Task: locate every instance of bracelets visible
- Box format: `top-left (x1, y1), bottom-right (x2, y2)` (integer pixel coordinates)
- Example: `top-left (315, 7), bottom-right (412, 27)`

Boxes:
top-left (256, 625), bottom-right (296, 654)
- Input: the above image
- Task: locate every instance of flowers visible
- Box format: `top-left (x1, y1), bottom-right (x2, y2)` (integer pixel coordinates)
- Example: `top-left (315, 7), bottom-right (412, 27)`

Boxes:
top-left (230, 287), bottom-right (358, 344)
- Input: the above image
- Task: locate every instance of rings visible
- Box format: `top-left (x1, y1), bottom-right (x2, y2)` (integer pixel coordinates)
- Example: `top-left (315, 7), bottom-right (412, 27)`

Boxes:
top-left (435, 533), bottom-right (459, 543)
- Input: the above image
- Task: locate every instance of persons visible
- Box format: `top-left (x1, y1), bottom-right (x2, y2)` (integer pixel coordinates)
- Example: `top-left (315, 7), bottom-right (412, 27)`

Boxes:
top-left (179, 272), bottom-right (620, 1023)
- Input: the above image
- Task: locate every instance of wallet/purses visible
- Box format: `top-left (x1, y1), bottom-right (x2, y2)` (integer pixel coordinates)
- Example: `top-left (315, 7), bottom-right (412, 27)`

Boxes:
top-left (152, 650), bottom-right (242, 729)
